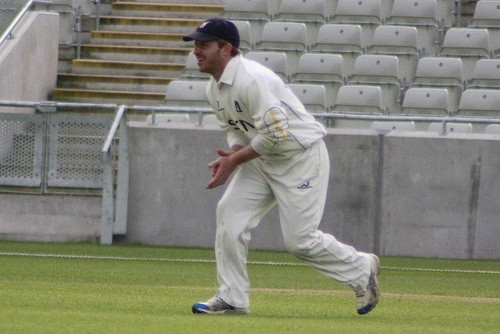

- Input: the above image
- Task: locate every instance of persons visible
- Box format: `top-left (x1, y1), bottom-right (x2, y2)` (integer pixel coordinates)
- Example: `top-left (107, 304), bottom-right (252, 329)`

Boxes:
top-left (181, 18), bottom-right (382, 315)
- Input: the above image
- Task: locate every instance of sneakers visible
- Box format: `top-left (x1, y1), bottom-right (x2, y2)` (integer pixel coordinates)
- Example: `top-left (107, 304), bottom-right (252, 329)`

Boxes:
top-left (349, 254), bottom-right (380, 315)
top-left (192, 295), bottom-right (249, 315)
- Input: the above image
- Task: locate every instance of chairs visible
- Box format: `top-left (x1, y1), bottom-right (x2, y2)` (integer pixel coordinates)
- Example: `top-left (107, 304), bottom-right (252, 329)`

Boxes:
top-left (32, 0), bottom-right (102, 72)
top-left (146, 0), bottom-right (500, 135)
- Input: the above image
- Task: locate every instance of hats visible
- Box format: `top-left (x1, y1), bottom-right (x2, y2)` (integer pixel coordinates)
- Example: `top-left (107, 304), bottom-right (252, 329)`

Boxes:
top-left (183, 18), bottom-right (240, 48)
